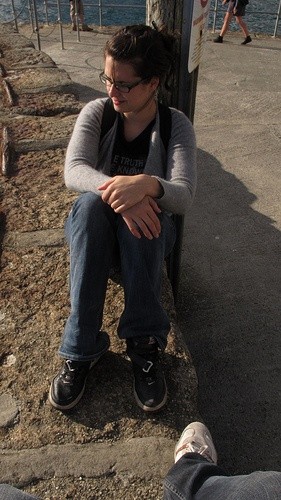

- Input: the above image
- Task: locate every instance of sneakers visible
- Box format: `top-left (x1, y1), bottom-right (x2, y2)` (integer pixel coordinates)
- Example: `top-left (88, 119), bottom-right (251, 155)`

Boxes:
top-left (49, 358), bottom-right (100, 408)
top-left (174, 422), bottom-right (217, 468)
top-left (127, 338), bottom-right (167, 412)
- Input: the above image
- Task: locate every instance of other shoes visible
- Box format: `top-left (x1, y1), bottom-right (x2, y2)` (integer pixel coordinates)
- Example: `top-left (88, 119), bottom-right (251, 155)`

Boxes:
top-left (82, 24), bottom-right (93, 31)
top-left (72, 27), bottom-right (81, 30)
top-left (214, 35), bottom-right (223, 43)
top-left (242, 36), bottom-right (252, 44)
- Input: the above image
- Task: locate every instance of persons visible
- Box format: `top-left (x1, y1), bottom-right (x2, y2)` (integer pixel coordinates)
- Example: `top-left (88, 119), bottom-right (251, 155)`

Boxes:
top-left (163, 422), bottom-right (280, 500)
top-left (49, 23), bottom-right (196, 411)
top-left (69, 0), bottom-right (92, 31)
top-left (212, 0), bottom-right (252, 45)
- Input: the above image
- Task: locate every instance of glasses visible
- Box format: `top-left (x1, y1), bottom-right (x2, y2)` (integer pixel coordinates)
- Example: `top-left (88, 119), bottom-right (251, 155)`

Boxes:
top-left (99, 72), bottom-right (147, 93)
